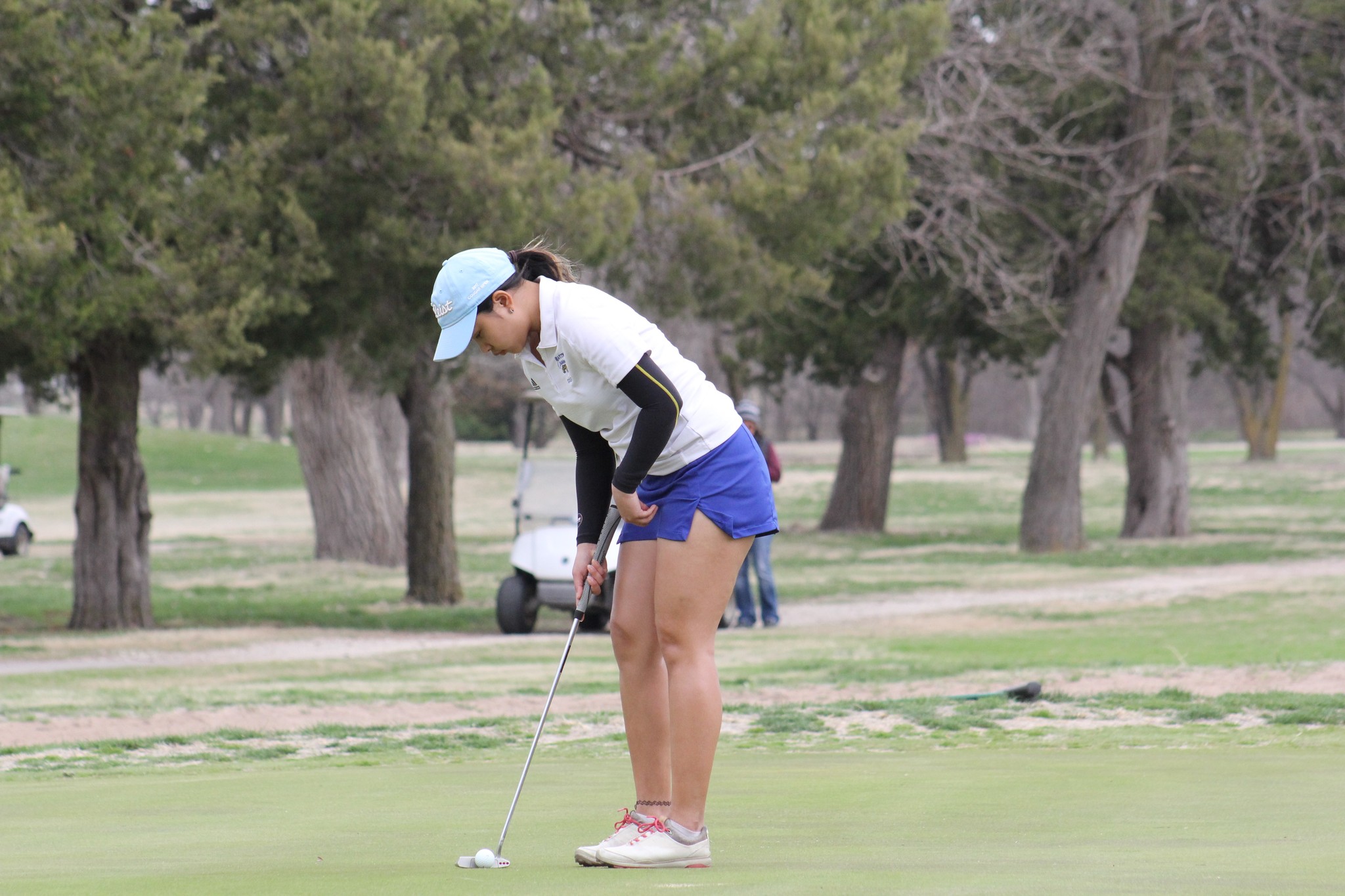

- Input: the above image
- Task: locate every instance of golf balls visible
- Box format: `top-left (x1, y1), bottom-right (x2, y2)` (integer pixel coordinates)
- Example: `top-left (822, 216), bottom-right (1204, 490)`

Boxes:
top-left (475, 849), bottom-right (495, 868)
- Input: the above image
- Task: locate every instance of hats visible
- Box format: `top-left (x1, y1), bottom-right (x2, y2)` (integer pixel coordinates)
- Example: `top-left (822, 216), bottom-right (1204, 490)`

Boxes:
top-left (735, 398), bottom-right (761, 426)
top-left (430, 247), bottom-right (516, 362)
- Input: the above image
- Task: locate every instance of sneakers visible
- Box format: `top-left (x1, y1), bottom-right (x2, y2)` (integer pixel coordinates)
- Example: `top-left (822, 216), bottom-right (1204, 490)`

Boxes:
top-left (596, 816), bottom-right (712, 868)
top-left (574, 807), bottom-right (656, 867)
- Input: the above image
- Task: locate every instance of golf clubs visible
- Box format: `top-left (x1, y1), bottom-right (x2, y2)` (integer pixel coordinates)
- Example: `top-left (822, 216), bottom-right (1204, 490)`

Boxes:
top-left (457, 505), bottom-right (622, 868)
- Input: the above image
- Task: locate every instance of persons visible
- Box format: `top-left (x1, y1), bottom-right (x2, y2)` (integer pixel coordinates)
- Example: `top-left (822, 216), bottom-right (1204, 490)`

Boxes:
top-left (735, 398), bottom-right (783, 628)
top-left (432, 248), bottom-right (778, 870)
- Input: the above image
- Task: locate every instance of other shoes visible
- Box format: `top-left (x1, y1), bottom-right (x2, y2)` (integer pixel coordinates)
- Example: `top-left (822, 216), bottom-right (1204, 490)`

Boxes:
top-left (762, 617), bottom-right (776, 627)
top-left (733, 620), bottom-right (752, 628)
